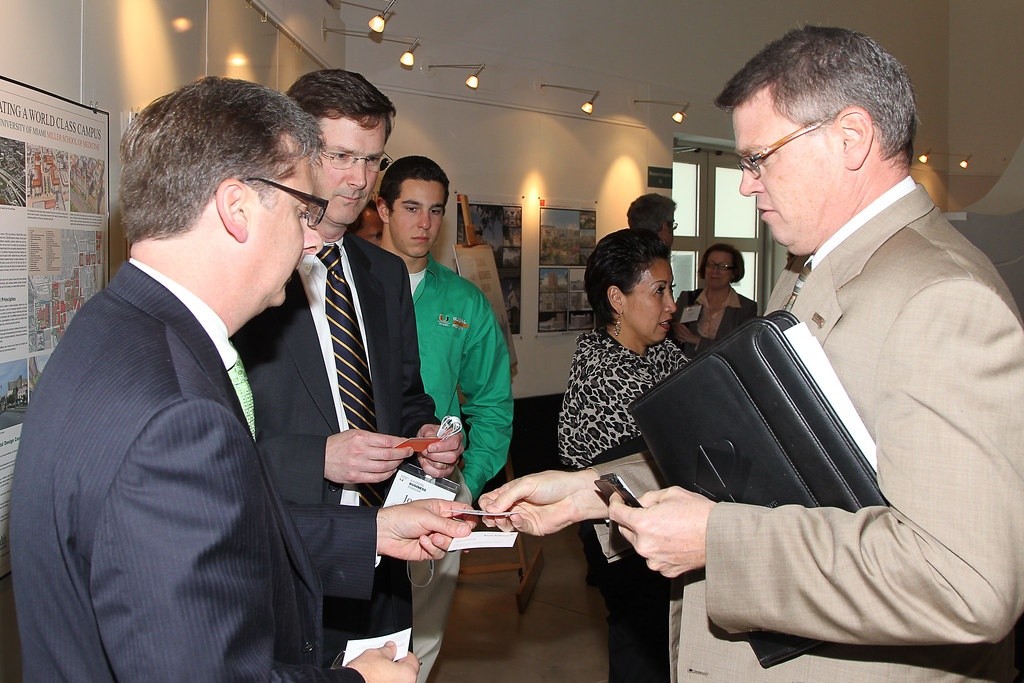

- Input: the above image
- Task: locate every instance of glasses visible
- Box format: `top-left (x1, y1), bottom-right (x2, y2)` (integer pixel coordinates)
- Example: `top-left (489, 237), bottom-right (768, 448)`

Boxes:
top-left (321, 150), bottom-right (393, 173)
top-left (659, 219), bottom-right (678, 230)
top-left (736, 110), bottom-right (840, 180)
top-left (704, 261), bottom-right (733, 271)
top-left (214, 178), bottom-right (330, 228)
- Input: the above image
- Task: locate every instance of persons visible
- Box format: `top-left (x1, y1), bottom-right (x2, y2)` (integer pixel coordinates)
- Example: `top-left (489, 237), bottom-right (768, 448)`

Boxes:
top-left (626, 193), bottom-right (677, 255)
top-left (7, 74), bottom-right (427, 682)
top-left (559, 228), bottom-right (695, 683)
top-left (668, 242), bottom-right (758, 360)
top-left (347, 200), bottom-right (382, 245)
top-left (477, 17), bottom-right (1024, 683)
top-left (344, 155), bottom-right (517, 683)
top-left (229, 67), bottom-right (467, 655)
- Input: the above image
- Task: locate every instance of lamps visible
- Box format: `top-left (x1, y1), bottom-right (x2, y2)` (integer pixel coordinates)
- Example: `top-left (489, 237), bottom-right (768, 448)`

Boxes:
top-left (322, 0), bottom-right (422, 66)
top-left (428, 64), bottom-right (485, 89)
top-left (542, 84), bottom-right (601, 114)
top-left (919, 147), bottom-right (973, 169)
top-left (633, 99), bottom-right (690, 123)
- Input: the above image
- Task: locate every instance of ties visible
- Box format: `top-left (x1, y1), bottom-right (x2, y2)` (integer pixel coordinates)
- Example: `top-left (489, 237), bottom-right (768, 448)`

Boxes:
top-left (782, 259), bottom-right (812, 314)
top-left (224, 340), bottom-right (256, 446)
top-left (316, 243), bottom-right (388, 508)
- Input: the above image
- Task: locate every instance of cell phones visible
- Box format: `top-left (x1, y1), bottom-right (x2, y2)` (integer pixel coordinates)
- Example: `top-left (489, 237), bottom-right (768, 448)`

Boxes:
top-left (593, 473), bottom-right (645, 511)
